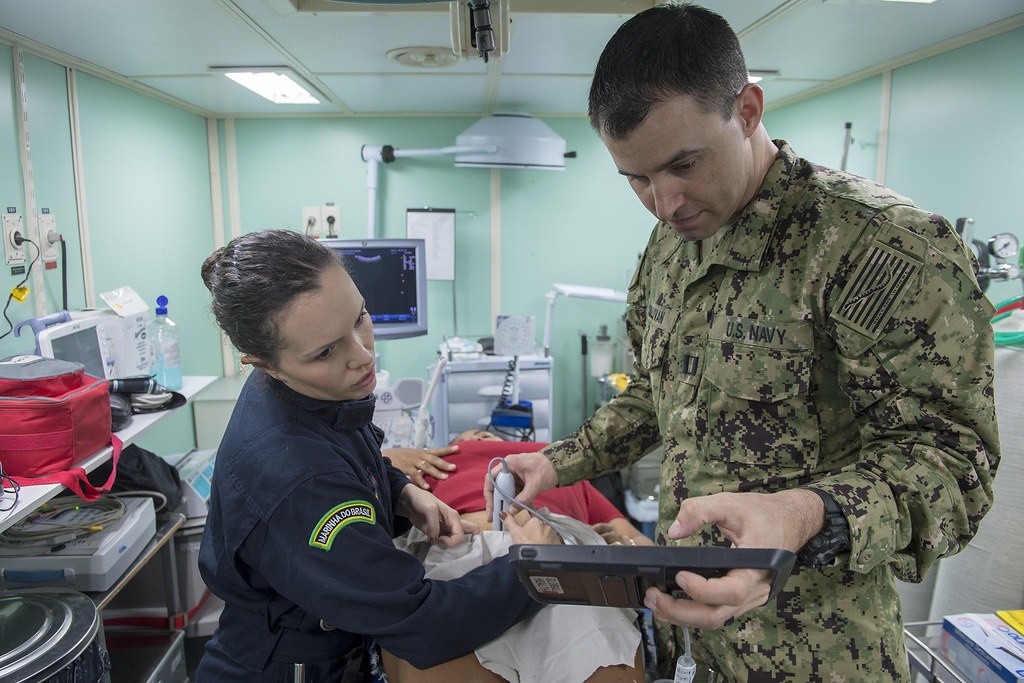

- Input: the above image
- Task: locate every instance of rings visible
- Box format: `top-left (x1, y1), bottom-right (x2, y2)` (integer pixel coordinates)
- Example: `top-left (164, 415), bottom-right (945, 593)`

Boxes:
top-left (614, 541), bottom-right (624, 546)
top-left (420, 460), bottom-right (426, 469)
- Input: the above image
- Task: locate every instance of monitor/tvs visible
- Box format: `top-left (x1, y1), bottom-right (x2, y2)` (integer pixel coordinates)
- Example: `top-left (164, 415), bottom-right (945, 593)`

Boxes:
top-left (315, 239), bottom-right (428, 340)
top-left (38, 316), bottom-right (115, 385)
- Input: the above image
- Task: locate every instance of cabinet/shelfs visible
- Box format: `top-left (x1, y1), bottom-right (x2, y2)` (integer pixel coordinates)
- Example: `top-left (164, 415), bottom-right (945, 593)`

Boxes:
top-left (194, 376), bottom-right (245, 452)
top-left (443, 354), bottom-right (553, 445)
top-left (84, 511), bottom-right (188, 683)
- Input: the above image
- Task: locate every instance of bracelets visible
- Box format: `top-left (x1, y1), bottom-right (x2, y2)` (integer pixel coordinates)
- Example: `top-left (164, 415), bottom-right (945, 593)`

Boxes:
top-left (624, 535), bottom-right (637, 546)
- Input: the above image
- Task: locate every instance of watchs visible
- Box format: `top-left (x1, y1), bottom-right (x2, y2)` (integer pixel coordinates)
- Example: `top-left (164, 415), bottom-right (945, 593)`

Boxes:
top-left (792, 487), bottom-right (850, 569)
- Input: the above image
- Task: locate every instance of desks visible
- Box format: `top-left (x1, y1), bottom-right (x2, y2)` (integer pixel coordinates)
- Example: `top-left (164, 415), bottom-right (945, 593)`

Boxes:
top-left (0, 376), bottom-right (219, 531)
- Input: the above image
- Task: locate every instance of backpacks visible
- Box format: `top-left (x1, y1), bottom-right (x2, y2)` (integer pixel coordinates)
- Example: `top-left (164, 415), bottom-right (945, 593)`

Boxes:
top-left (0, 354), bottom-right (123, 503)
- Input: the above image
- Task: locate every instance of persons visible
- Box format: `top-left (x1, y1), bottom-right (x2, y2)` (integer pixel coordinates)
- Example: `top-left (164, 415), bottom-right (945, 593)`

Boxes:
top-left (379, 428), bottom-right (662, 683)
top-left (485, 1), bottom-right (1003, 683)
top-left (194, 230), bottom-right (561, 683)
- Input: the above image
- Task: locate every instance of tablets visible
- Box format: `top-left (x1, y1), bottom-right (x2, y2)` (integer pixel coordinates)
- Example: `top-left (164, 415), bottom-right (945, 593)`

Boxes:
top-left (508, 544), bottom-right (794, 606)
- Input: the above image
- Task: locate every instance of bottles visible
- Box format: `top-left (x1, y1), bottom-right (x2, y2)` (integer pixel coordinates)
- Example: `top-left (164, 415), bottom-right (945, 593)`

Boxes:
top-left (148, 296), bottom-right (183, 390)
